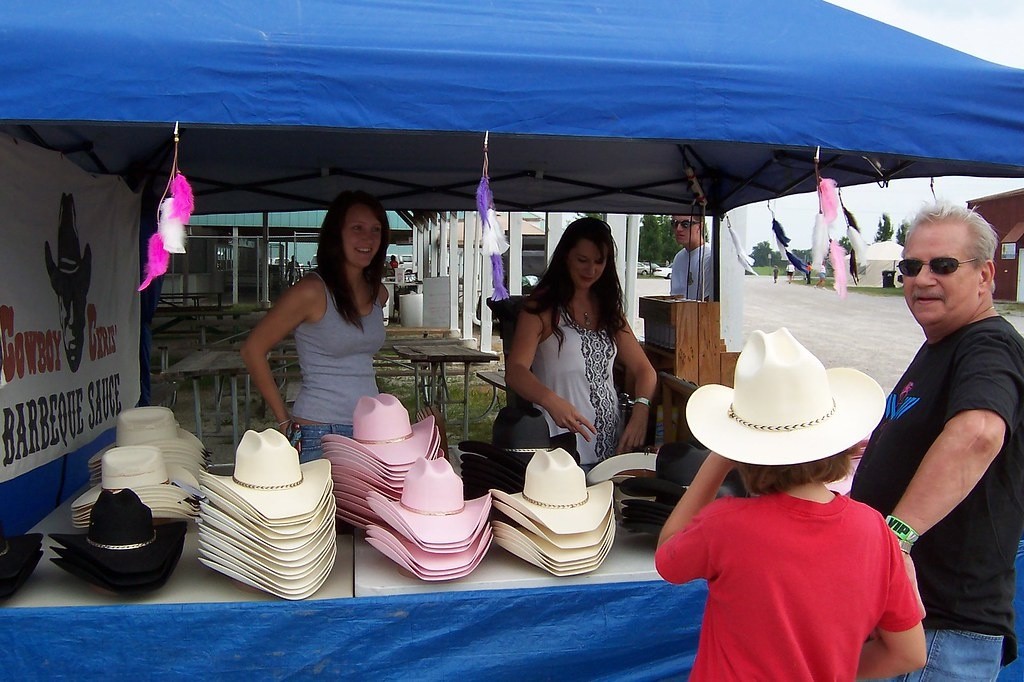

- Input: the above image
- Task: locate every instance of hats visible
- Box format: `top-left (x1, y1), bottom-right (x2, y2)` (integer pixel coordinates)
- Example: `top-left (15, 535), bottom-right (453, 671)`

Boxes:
top-left (488, 447), bottom-right (617, 579)
top-left (47, 407), bottom-right (214, 594)
top-left (458, 405), bottom-right (581, 527)
top-left (0, 522), bottom-right (38, 601)
top-left (365, 457), bottom-right (493, 582)
top-left (587, 446), bottom-right (662, 527)
top-left (321, 393), bottom-right (445, 532)
top-left (620, 439), bottom-right (750, 536)
top-left (685, 326), bottom-right (887, 465)
top-left (197, 426), bottom-right (336, 600)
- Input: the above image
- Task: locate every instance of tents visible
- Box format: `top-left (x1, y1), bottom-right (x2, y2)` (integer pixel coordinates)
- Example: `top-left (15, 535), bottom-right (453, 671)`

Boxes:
top-left (0, 0), bottom-right (1024, 436)
top-left (833, 239), bottom-right (904, 288)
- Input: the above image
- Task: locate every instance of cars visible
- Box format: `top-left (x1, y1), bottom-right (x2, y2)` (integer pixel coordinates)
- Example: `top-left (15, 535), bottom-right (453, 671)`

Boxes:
top-left (652, 263), bottom-right (673, 279)
top-left (638, 262), bottom-right (659, 276)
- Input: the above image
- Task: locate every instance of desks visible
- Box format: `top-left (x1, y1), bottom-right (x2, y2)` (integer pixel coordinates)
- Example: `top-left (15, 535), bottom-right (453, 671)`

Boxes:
top-left (159, 348), bottom-right (248, 454)
top-left (392, 343), bottom-right (501, 442)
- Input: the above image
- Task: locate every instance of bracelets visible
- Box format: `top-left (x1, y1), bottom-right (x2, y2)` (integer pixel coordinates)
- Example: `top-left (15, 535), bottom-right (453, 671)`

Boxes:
top-left (885, 515), bottom-right (919, 554)
top-left (278, 419), bottom-right (291, 426)
top-left (634, 396), bottom-right (651, 408)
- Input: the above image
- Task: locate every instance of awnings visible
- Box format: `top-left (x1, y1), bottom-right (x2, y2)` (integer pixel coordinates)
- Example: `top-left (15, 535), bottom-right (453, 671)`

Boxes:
top-left (1002, 222), bottom-right (1024, 243)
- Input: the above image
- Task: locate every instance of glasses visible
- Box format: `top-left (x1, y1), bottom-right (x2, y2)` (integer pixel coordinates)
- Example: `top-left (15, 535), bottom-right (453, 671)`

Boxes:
top-left (671, 218), bottom-right (700, 229)
top-left (896, 257), bottom-right (981, 276)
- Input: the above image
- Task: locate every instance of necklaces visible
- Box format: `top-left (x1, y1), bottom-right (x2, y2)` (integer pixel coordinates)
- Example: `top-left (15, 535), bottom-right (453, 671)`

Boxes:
top-left (575, 307), bottom-right (601, 328)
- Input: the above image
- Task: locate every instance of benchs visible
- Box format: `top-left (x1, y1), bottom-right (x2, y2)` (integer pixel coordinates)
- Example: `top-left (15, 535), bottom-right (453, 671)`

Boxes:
top-left (375, 369), bottom-right (468, 405)
top-left (476, 371), bottom-right (506, 422)
top-left (286, 380), bottom-right (302, 402)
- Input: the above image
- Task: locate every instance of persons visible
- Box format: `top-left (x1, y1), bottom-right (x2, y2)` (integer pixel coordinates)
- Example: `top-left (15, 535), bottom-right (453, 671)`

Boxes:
top-left (390, 255), bottom-right (398, 268)
top-left (285, 255), bottom-right (300, 287)
top-left (505, 217), bottom-right (657, 465)
top-left (774, 266), bottom-right (778, 283)
top-left (665, 258), bottom-right (669, 267)
top-left (654, 327), bottom-right (926, 682)
top-left (240, 189), bottom-right (389, 464)
top-left (849, 201), bottom-right (1024, 682)
top-left (807, 262), bottom-right (812, 284)
top-left (786, 261), bottom-right (795, 284)
top-left (814, 260), bottom-right (827, 290)
top-left (307, 261), bottom-right (311, 271)
top-left (669, 215), bottom-right (711, 298)
